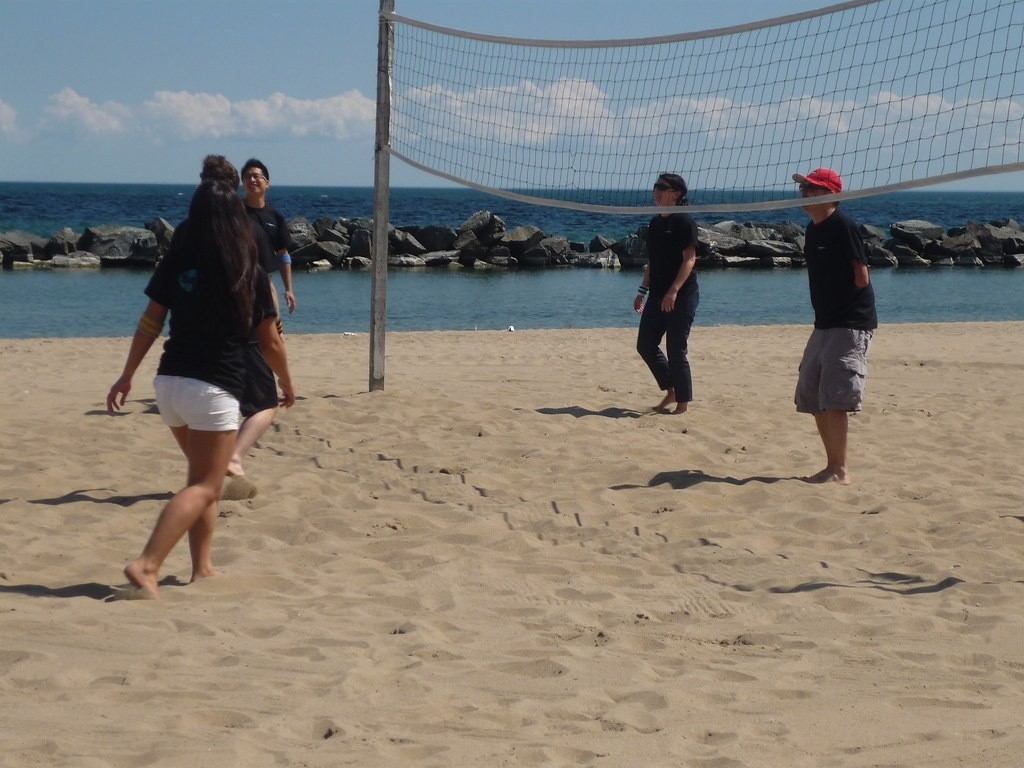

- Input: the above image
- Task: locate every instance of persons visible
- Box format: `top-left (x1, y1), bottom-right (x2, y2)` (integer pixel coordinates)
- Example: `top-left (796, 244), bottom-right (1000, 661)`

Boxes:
top-left (633, 173), bottom-right (700, 415)
top-left (107, 154), bottom-right (296, 600)
top-left (792, 168), bottom-right (878, 485)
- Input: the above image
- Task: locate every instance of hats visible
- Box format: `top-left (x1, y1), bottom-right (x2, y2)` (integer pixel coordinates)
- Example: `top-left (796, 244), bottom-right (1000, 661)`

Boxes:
top-left (793, 168), bottom-right (842, 194)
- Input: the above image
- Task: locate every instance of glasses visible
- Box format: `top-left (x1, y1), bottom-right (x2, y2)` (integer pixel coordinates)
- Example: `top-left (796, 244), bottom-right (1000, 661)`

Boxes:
top-left (653, 182), bottom-right (677, 193)
top-left (799, 182), bottom-right (827, 192)
top-left (240, 173), bottom-right (268, 182)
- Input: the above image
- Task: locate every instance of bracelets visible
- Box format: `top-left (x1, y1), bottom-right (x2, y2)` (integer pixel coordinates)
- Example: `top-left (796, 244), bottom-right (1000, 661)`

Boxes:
top-left (637, 284), bottom-right (649, 296)
